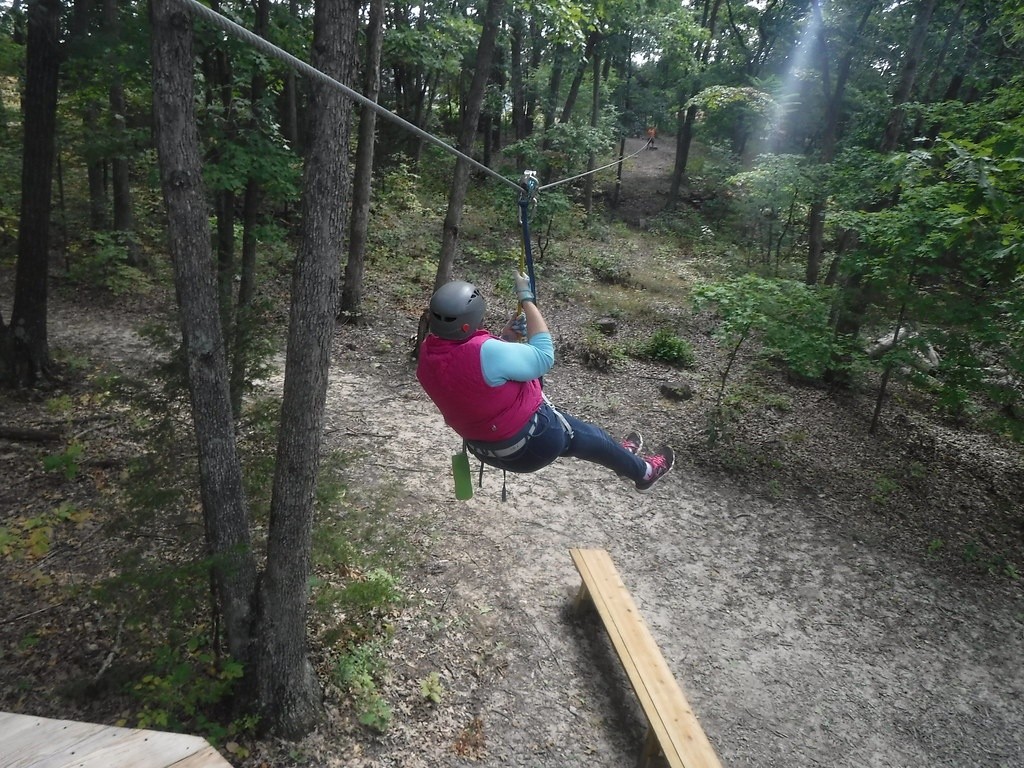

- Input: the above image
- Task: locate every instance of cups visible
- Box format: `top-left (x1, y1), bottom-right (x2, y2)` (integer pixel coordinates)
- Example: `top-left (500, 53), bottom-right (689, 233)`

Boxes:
top-left (452, 452), bottom-right (473, 500)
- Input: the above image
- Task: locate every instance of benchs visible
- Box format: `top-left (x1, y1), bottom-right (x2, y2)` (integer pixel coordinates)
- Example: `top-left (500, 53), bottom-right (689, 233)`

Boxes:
top-left (569, 548), bottom-right (722, 768)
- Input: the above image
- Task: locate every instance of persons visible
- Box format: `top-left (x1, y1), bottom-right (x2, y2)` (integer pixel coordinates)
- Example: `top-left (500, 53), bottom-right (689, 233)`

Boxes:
top-left (416, 271), bottom-right (675, 494)
top-left (646, 127), bottom-right (654, 149)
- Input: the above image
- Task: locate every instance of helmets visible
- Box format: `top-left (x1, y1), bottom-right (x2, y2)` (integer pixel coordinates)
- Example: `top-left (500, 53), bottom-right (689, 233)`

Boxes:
top-left (427, 281), bottom-right (487, 340)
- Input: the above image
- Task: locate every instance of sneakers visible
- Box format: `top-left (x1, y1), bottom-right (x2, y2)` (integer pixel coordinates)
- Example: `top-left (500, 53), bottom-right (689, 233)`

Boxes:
top-left (614, 430), bottom-right (643, 480)
top-left (635, 445), bottom-right (675, 493)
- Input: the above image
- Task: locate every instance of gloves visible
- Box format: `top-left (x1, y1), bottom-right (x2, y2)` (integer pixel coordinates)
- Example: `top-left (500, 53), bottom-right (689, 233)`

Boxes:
top-left (499, 311), bottom-right (527, 342)
top-left (512, 270), bottom-right (535, 305)
